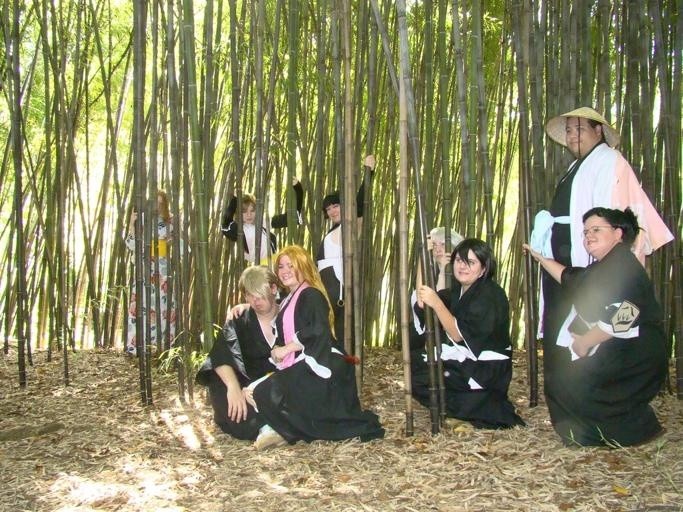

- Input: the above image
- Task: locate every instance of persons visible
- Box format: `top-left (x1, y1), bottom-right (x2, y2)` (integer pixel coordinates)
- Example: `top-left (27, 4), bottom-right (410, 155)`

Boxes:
top-left (409, 227), bottom-right (528, 429)
top-left (530, 107), bottom-right (675, 371)
top-left (318, 155), bottom-right (375, 356)
top-left (195, 244), bottom-right (386, 451)
top-left (221, 176), bottom-right (302, 305)
top-left (125, 191), bottom-right (191, 356)
top-left (522, 207), bottom-right (669, 450)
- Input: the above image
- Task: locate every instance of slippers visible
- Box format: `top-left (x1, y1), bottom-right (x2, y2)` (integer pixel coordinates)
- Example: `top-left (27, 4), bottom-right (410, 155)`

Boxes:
top-left (254, 433), bottom-right (288, 450)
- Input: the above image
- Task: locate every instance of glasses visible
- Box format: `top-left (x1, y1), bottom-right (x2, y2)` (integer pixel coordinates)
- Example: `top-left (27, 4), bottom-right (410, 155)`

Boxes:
top-left (582, 227), bottom-right (598, 236)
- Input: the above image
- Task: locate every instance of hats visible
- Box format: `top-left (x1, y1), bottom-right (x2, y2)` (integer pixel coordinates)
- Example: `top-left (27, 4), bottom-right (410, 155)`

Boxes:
top-left (546, 107), bottom-right (620, 149)
